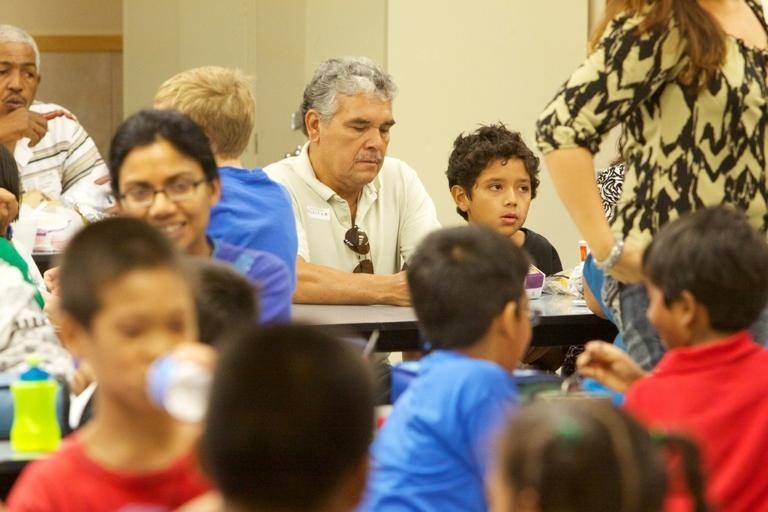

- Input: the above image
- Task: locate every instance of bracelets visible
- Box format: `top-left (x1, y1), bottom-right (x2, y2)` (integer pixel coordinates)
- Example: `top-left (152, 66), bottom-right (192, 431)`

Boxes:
top-left (593, 239), bottom-right (623, 271)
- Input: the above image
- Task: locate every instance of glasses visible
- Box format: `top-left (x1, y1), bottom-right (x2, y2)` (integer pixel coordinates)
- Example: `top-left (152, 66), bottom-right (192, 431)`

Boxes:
top-left (344, 225), bottom-right (374, 274)
top-left (117, 174), bottom-right (212, 210)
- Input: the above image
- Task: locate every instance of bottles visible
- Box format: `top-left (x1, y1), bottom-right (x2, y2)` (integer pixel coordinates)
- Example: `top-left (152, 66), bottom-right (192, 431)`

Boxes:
top-left (9, 355), bottom-right (62, 454)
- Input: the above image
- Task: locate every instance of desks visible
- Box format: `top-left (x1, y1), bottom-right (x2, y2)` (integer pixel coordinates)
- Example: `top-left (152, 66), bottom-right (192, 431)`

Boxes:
top-left (291, 293), bottom-right (618, 353)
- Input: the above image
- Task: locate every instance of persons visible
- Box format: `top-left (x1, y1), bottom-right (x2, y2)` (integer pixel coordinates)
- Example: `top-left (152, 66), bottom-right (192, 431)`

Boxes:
top-left (404, 122), bottom-right (564, 373)
top-left (171, 318), bottom-right (374, 512)
top-left (480, 393), bottom-right (708, 511)
top-left (261, 55), bottom-right (442, 308)
top-left (534, 1), bottom-right (768, 373)
top-left (1, 22), bottom-right (116, 254)
top-left (574, 207), bottom-right (768, 510)
top-left (41, 256), bottom-right (262, 429)
top-left (0, 188), bottom-right (18, 226)
top-left (350, 226), bottom-right (534, 511)
top-left (1, 260), bottom-right (76, 439)
top-left (0, 218), bottom-right (224, 512)
top-left (0, 144), bottom-right (45, 312)
top-left (151, 67), bottom-right (298, 325)
top-left (109, 106), bottom-right (291, 333)
